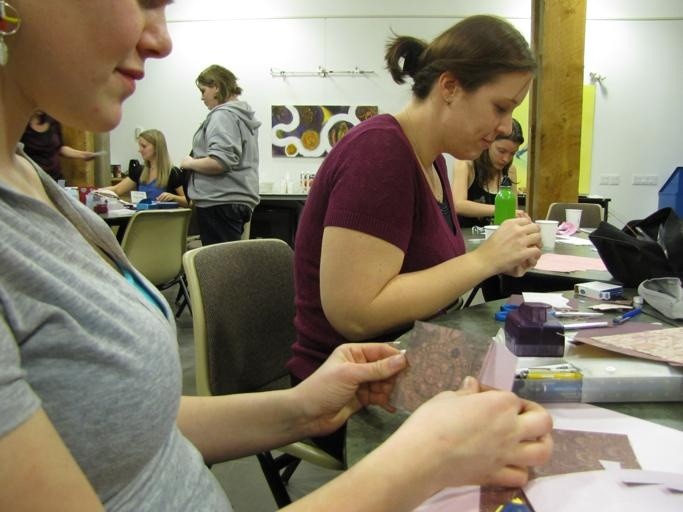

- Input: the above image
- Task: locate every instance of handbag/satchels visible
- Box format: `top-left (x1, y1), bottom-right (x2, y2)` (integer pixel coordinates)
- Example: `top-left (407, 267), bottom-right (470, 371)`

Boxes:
top-left (587, 205), bottom-right (682, 288)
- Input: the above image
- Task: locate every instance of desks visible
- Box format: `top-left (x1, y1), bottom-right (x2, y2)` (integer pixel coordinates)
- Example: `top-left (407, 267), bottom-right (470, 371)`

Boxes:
top-left (347, 285), bottom-right (680, 512)
top-left (454, 225), bottom-right (615, 311)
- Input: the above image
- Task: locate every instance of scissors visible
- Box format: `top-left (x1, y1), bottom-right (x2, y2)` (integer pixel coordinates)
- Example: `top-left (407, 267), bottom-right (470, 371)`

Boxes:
top-left (495, 303), bottom-right (604, 322)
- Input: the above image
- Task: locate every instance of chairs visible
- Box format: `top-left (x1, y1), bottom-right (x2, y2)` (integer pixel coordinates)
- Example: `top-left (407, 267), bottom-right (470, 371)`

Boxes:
top-left (544, 202), bottom-right (605, 228)
top-left (54, 163), bottom-right (205, 333)
top-left (179, 238), bottom-right (348, 512)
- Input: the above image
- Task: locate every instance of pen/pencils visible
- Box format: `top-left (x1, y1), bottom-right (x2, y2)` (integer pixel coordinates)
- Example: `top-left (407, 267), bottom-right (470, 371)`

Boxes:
top-left (563, 321), bottom-right (609, 330)
top-left (512, 370), bottom-right (583, 403)
top-left (613, 307), bottom-right (642, 325)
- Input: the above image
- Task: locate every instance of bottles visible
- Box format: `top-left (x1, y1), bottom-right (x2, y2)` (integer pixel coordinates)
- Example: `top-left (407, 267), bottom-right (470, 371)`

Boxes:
top-left (494, 175), bottom-right (516, 225)
top-left (128, 160), bottom-right (138, 171)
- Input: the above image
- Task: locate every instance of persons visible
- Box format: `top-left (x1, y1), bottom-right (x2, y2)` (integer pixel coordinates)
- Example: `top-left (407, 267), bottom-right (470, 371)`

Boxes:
top-left (285, 12), bottom-right (544, 463)
top-left (180, 66), bottom-right (261, 246)
top-left (1, 0), bottom-right (552, 511)
top-left (93, 128), bottom-right (193, 251)
top-left (18, 110), bottom-right (100, 182)
top-left (450, 117), bottom-right (523, 226)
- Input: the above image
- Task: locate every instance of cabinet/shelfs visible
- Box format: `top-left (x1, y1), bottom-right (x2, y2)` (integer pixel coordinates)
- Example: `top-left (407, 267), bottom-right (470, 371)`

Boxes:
top-left (243, 193), bottom-right (312, 254)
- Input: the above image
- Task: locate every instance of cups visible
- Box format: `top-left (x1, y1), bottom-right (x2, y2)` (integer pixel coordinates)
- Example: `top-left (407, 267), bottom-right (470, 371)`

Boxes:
top-left (483, 224), bottom-right (498, 239)
top-left (110, 164), bottom-right (121, 178)
top-left (565, 209), bottom-right (582, 232)
top-left (65, 184), bottom-right (107, 213)
top-left (534, 221), bottom-right (558, 251)
